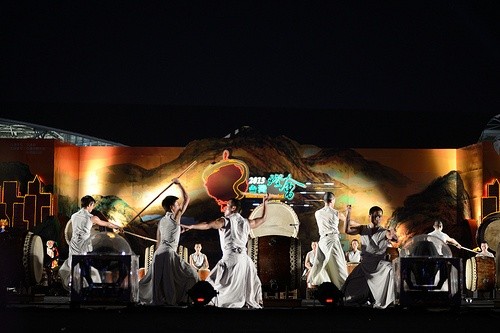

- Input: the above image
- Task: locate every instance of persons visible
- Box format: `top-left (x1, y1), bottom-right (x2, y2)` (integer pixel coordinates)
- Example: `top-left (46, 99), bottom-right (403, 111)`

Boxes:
top-left (426, 220), bottom-right (462, 290)
top-left (305, 241), bottom-right (318, 288)
top-left (189, 243), bottom-right (210, 271)
top-left (35, 214), bottom-right (63, 290)
top-left (307, 192), bottom-right (349, 291)
top-left (476, 240), bottom-right (494, 258)
top-left (338, 203), bottom-right (398, 308)
top-left (64, 193), bottom-right (125, 289)
top-left (178, 192), bottom-right (269, 308)
top-left (344, 239), bottom-right (362, 263)
top-left (151, 178), bottom-right (200, 304)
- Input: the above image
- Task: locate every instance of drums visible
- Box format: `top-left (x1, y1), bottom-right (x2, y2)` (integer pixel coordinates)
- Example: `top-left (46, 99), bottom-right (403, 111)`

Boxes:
top-left (0, 230), bottom-right (44, 286)
top-left (246, 235), bottom-right (301, 293)
top-left (248, 201), bottom-right (303, 240)
top-left (199, 268), bottom-right (210, 281)
top-left (138, 267), bottom-right (145, 281)
top-left (104, 271), bottom-right (128, 288)
top-left (144, 242), bottom-right (188, 276)
top-left (477, 211), bottom-right (500, 253)
top-left (346, 261), bottom-right (361, 276)
top-left (466, 255), bottom-right (497, 292)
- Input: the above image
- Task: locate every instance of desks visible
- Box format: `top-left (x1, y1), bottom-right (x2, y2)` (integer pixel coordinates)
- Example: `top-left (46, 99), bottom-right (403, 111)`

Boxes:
top-left (71, 254), bottom-right (131, 306)
top-left (399, 256), bottom-right (459, 308)
